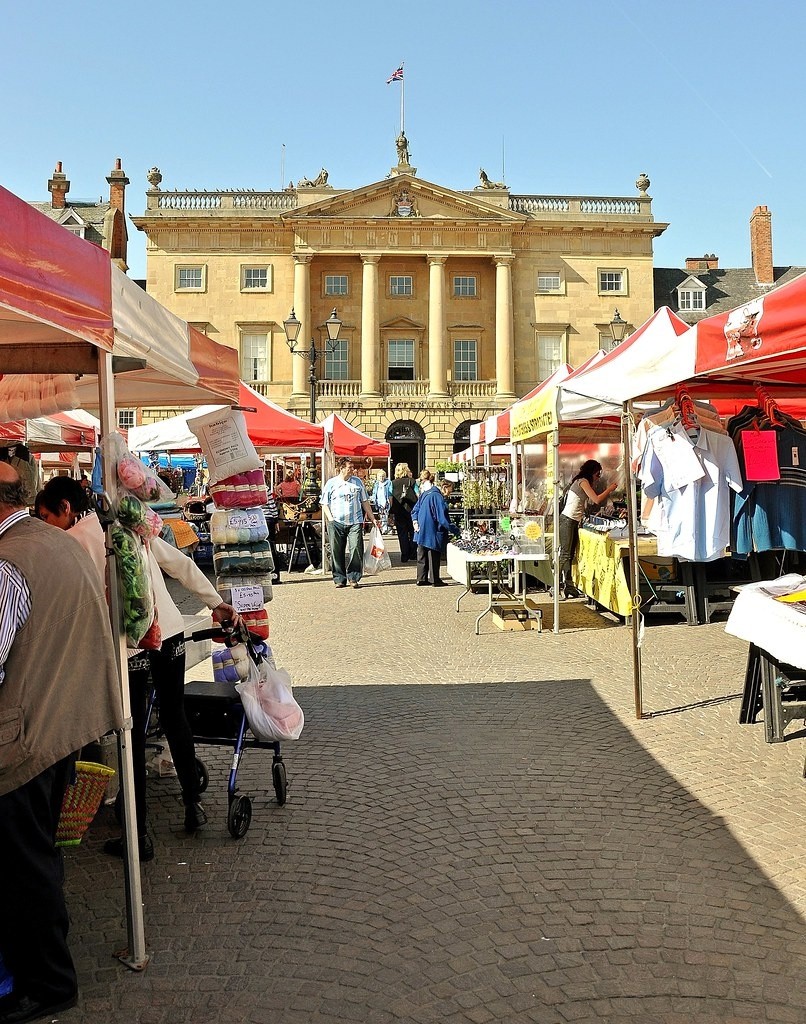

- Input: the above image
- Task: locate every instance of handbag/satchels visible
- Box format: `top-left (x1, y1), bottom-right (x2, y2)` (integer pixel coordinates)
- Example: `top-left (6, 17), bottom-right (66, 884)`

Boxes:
top-left (400, 479), bottom-right (417, 506)
top-left (558, 484), bottom-right (570, 516)
top-left (234, 650), bottom-right (304, 742)
top-left (363, 525), bottom-right (391, 575)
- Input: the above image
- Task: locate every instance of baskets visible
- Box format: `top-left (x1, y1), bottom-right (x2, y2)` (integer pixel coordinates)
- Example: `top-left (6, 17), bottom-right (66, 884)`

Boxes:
top-left (53, 761), bottom-right (115, 847)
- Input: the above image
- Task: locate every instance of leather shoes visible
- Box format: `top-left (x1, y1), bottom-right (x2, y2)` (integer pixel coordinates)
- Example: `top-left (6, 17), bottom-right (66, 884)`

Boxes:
top-left (185, 804), bottom-right (208, 829)
top-left (0, 992), bottom-right (78, 1024)
top-left (104, 835), bottom-right (154, 862)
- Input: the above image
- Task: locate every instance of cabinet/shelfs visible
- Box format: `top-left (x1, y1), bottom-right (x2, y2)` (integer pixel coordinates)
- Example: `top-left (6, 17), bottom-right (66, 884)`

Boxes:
top-left (438, 470), bottom-right (464, 541)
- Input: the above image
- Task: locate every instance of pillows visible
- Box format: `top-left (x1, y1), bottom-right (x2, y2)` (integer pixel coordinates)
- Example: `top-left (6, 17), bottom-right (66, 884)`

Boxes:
top-left (187, 405), bottom-right (267, 487)
top-left (0, 374), bottom-right (80, 423)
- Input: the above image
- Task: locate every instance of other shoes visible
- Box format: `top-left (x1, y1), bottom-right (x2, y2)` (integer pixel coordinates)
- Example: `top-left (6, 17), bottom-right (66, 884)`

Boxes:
top-left (417, 581), bottom-right (432, 586)
top-left (350, 579), bottom-right (358, 587)
top-left (335, 582), bottom-right (345, 588)
top-left (433, 582), bottom-right (448, 587)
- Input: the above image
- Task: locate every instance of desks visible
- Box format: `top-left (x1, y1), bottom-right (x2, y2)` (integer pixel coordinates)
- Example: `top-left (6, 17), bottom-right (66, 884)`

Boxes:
top-left (725, 584), bottom-right (806, 779)
top-left (283, 520), bottom-right (323, 574)
top-left (446, 543), bottom-right (512, 594)
top-left (569, 529), bottom-right (761, 626)
top-left (456, 554), bottom-right (550, 635)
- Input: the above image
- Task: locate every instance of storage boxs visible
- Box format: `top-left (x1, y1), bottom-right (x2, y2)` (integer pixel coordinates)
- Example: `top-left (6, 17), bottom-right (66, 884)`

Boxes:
top-left (493, 598), bottom-right (542, 621)
top-left (496, 510), bottom-right (545, 554)
top-left (492, 609), bottom-right (543, 632)
top-left (180, 615), bottom-right (211, 671)
top-left (99, 734), bottom-right (120, 805)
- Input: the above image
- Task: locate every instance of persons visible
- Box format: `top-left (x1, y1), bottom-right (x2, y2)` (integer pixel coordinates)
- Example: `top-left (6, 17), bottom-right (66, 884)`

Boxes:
top-left (277, 472), bottom-right (302, 498)
top-left (255, 475), bottom-right (280, 547)
top-left (30, 475), bottom-right (244, 859)
top-left (407, 469), bottom-right (433, 499)
top-left (410, 478), bottom-right (463, 588)
top-left (410, 196), bottom-right (424, 217)
top-left (508, 479), bottom-right (538, 514)
top-left (386, 194), bottom-right (400, 216)
top-left (552, 458), bottom-right (619, 600)
top-left (0, 441), bottom-right (124, 1024)
top-left (370, 470), bottom-right (392, 535)
top-left (320, 456), bottom-right (379, 588)
top-left (390, 462), bottom-right (418, 563)
top-left (394, 130), bottom-right (410, 165)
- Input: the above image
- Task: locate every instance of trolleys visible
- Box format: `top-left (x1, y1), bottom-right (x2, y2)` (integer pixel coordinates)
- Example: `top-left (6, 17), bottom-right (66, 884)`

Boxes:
top-left (144, 618), bottom-right (288, 840)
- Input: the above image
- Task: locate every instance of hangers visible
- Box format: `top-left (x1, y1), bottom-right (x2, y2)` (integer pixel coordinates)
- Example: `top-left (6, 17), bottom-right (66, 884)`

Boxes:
top-left (674, 383), bottom-right (700, 431)
top-left (755, 386), bottom-right (787, 429)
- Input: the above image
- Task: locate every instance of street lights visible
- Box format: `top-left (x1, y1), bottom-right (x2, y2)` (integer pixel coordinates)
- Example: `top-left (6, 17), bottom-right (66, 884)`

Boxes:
top-left (282, 306), bottom-right (344, 509)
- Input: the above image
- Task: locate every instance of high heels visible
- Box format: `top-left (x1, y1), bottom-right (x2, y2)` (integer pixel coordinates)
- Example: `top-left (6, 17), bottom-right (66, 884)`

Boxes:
top-left (559, 583), bottom-right (565, 595)
top-left (564, 584), bottom-right (585, 599)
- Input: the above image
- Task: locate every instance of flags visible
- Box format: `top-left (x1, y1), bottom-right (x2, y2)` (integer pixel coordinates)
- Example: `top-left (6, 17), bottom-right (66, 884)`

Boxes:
top-left (386, 64), bottom-right (404, 85)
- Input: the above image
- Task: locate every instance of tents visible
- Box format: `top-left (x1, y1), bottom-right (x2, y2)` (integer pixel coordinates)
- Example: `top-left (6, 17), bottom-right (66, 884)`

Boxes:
top-left (1, 183), bottom-right (243, 971)
top-left (265, 412), bottom-right (391, 491)
top-left (445, 270), bottom-right (805, 779)
top-left (127, 379), bottom-right (334, 576)
top-left (0, 405), bottom-right (129, 484)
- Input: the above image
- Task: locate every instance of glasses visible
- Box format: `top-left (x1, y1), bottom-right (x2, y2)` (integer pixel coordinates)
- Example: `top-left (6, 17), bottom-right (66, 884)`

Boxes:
top-left (595, 471), bottom-right (600, 477)
top-left (340, 456), bottom-right (353, 466)
